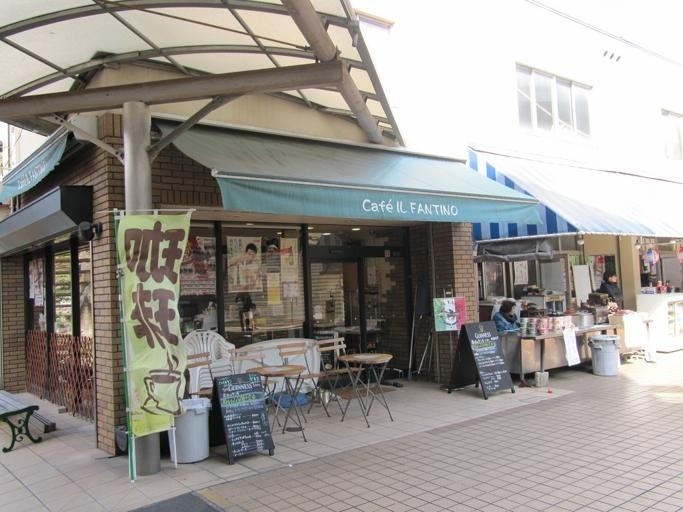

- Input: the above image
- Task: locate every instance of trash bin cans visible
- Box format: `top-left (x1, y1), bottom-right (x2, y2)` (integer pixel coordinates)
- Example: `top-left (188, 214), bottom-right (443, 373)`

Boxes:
top-left (588, 335), bottom-right (620, 376)
top-left (168, 397), bottom-right (212, 464)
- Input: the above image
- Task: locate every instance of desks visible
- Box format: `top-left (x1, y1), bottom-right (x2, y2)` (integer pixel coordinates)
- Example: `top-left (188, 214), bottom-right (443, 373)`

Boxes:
top-left (339, 352), bottom-right (396, 427)
top-left (505, 322), bottom-right (619, 382)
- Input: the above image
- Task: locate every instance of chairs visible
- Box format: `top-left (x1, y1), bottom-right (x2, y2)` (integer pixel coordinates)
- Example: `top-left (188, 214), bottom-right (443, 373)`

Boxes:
top-left (183, 330), bottom-right (364, 440)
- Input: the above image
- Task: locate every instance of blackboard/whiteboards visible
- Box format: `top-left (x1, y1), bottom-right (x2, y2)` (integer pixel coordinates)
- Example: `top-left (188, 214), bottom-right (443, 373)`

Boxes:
top-left (464, 321), bottom-right (513, 393)
top-left (214, 372), bottom-right (275, 457)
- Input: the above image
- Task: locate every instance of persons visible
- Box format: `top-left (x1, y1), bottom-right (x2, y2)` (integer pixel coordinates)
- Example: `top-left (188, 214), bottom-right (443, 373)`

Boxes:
top-left (493, 300), bottom-right (520, 332)
top-left (236, 292), bottom-right (260, 331)
top-left (599, 271), bottom-right (623, 308)
top-left (266, 242), bottom-right (278, 260)
top-left (228, 243), bottom-right (260, 286)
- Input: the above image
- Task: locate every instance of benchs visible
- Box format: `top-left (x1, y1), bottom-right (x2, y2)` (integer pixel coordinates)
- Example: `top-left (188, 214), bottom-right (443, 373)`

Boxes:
top-left (0, 390), bottom-right (43, 452)
